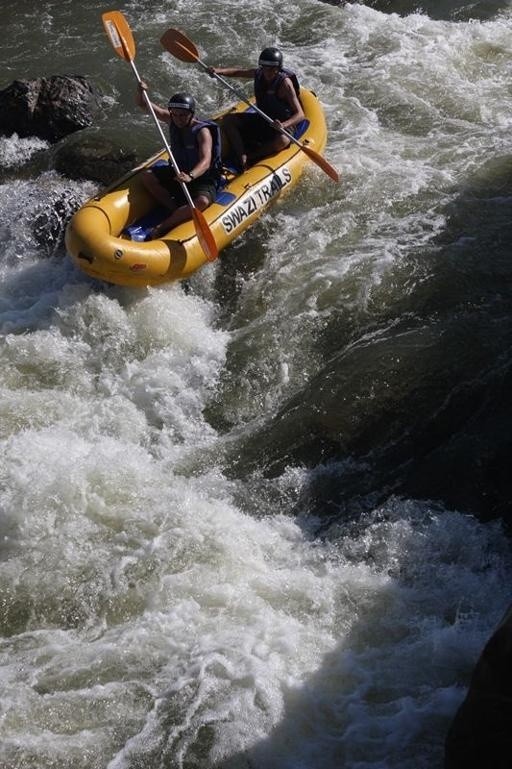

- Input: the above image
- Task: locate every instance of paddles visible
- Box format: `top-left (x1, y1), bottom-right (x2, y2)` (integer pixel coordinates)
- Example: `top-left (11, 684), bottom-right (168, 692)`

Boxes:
top-left (160, 29), bottom-right (340, 182)
top-left (103, 10), bottom-right (217, 262)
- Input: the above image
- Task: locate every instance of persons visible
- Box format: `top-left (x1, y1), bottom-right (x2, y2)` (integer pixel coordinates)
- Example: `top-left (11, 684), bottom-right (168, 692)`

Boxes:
top-left (206, 47), bottom-right (306, 178)
top-left (125, 81), bottom-right (222, 242)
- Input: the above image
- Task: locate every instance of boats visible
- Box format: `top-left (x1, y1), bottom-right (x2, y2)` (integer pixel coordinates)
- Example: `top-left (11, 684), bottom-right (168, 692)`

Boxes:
top-left (64, 86), bottom-right (329, 290)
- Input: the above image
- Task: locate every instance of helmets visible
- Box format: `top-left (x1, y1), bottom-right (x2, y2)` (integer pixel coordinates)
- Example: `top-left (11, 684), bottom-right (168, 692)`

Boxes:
top-left (167, 93), bottom-right (195, 116)
top-left (258, 48), bottom-right (282, 75)
